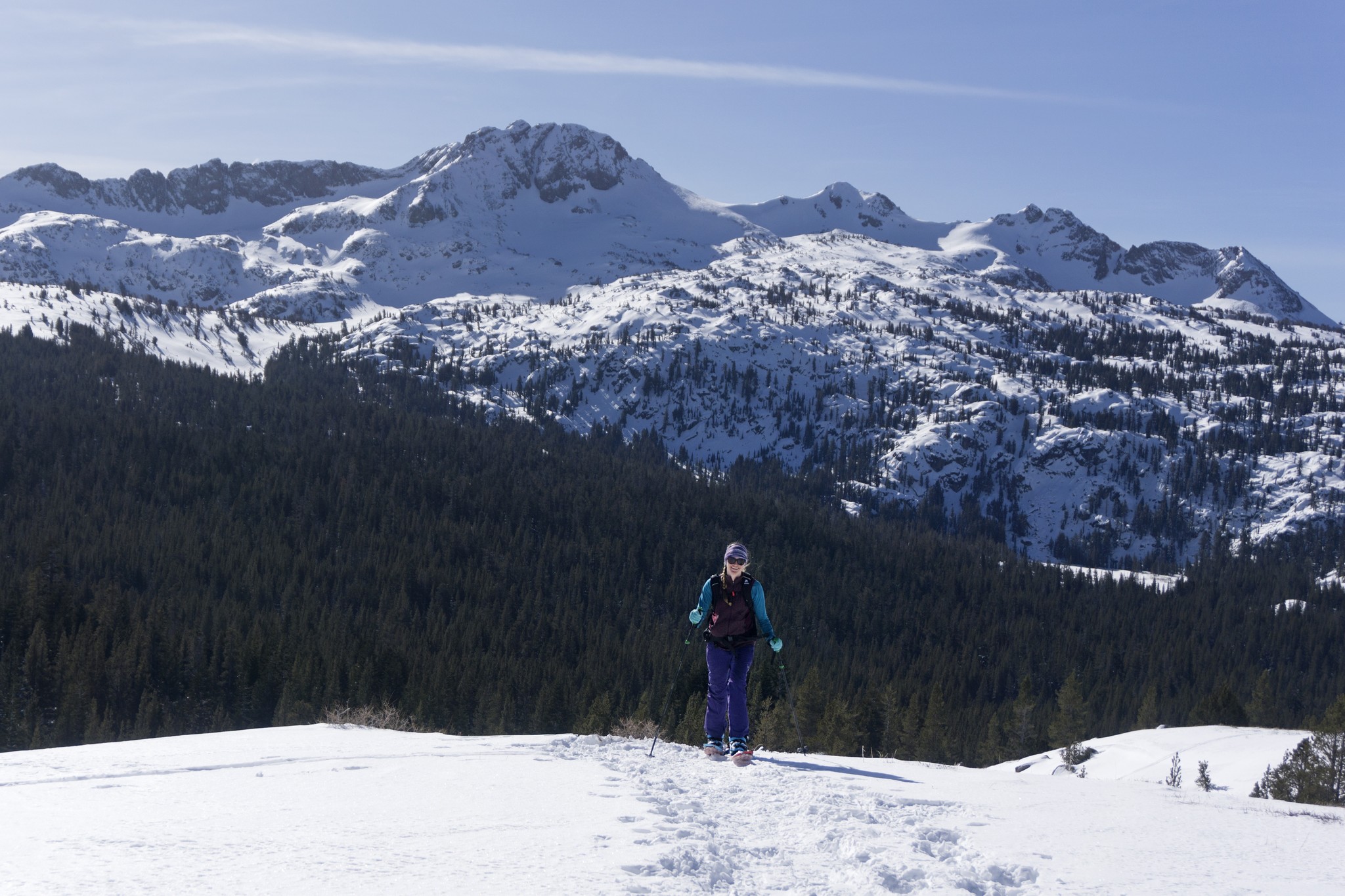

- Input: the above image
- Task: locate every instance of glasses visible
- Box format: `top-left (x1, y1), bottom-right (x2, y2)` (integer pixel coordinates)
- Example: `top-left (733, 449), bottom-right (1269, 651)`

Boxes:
top-left (726, 556), bottom-right (746, 566)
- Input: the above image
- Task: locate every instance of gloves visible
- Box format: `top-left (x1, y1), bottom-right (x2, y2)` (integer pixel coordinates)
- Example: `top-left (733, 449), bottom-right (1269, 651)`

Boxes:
top-left (689, 609), bottom-right (703, 624)
top-left (768, 637), bottom-right (783, 652)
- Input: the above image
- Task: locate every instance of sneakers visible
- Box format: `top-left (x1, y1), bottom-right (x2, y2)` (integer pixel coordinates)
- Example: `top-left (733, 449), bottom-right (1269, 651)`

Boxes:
top-left (728, 734), bottom-right (749, 755)
top-left (706, 733), bottom-right (724, 750)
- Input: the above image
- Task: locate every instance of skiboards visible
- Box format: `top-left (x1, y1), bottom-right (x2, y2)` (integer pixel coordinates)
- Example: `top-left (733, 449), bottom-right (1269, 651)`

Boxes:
top-left (703, 744), bottom-right (754, 759)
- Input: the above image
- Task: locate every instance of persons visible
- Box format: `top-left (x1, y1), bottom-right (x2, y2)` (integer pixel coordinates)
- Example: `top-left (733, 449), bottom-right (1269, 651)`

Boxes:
top-left (686, 540), bottom-right (784, 765)
